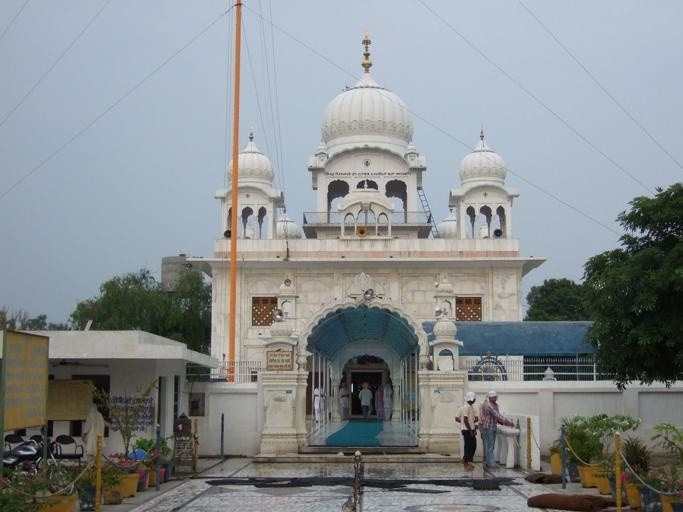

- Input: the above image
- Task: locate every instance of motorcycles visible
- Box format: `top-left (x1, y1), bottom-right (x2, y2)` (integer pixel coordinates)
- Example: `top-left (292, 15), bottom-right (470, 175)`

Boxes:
top-left (2, 436), bottom-right (75, 496)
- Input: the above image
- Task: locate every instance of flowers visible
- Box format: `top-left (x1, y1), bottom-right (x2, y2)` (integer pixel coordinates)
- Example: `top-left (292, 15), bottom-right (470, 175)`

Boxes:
top-left (616, 460), bottom-right (682, 497)
top-left (106, 436), bottom-right (171, 478)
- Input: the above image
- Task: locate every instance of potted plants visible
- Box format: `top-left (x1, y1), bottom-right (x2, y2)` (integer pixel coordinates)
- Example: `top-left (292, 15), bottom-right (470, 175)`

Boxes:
top-left (102, 467), bottom-right (124, 505)
top-left (549, 412), bottom-right (649, 498)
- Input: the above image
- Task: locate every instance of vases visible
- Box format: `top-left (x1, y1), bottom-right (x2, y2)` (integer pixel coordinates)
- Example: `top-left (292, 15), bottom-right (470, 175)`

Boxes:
top-left (624, 482), bottom-right (682, 511)
top-left (119, 467), bottom-right (166, 498)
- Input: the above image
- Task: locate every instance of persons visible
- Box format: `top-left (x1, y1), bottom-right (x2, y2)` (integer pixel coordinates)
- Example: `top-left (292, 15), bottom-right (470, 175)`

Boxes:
top-left (375, 385), bottom-right (384, 418)
top-left (339, 380), bottom-right (350, 417)
top-left (455, 390), bottom-right (514, 469)
top-left (359, 382), bottom-right (373, 418)
top-left (82, 403), bottom-right (105, 468)
top-left (382, 382), bottom-right (393, 421)
top-left (314, 384), bottom-right (325, 422)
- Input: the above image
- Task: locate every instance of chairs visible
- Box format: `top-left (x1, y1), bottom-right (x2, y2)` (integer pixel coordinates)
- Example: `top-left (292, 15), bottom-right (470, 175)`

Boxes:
top-left (3, 428), bottom-right (85, 473)
